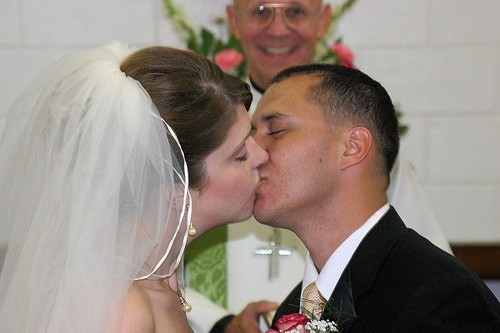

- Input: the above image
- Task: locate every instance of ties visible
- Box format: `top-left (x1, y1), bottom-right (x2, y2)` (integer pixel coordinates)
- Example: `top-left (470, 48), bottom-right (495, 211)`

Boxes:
top-left (300, 283), bottom-right (323, 321)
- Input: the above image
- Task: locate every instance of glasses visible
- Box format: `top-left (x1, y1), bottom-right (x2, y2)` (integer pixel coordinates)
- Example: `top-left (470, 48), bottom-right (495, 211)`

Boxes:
top-left (235, 2), bottom-right (323, 31)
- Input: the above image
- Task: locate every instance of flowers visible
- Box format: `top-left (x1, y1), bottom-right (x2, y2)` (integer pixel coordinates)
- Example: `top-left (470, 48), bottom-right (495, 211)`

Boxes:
top-left (265, 312), bottom-right (340, 333)
top-left (162, 0), bottom-right (356, 78)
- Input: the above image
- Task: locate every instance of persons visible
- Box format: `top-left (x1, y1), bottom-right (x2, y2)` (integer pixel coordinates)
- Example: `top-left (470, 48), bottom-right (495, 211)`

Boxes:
top-left (244, 64), bottom-right (500, 333)
top-left (0, 40), bottom-right (269, 333)
top-left (178, 0), bottom-right (454, 333)
